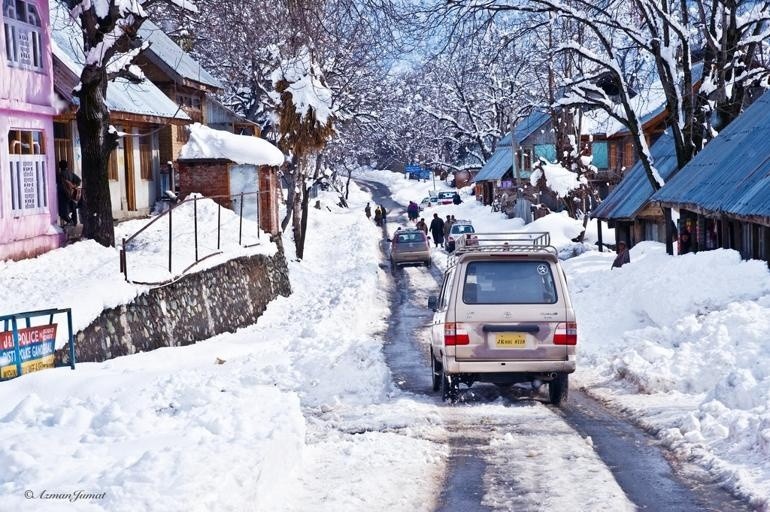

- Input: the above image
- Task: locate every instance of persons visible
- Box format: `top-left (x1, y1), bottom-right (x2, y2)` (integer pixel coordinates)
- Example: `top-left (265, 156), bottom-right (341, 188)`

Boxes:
top-left (57, 160), bottom-right (82, 226)
top-left (365, 201), bottom-right (372, 218)
top-left (452, 192), bottom-right (461, 205)
top-left (381, 205), bottom-right (388, 221)
top-left (678, 232), bottom-right (694, 255)
top-left (407, 200), bottom-right (456, 248)
top-left (609, 240), bottom-right (630, 270)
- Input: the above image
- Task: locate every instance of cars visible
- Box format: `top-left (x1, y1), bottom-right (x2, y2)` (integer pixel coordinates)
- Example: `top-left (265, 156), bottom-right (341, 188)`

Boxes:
top-left (418, 191), bottom-right (460, 211)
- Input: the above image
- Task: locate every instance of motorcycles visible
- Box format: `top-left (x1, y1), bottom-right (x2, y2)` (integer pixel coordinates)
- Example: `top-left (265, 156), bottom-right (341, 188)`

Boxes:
top-left (492, 194), bottom-right (501, 211)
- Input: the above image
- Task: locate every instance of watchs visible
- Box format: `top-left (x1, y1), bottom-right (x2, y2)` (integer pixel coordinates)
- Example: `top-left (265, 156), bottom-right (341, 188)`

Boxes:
top-left (375, 207), bottom-right (382, 226)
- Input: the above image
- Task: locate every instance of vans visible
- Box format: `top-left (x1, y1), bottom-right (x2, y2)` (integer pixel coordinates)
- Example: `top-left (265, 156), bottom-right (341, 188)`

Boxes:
top-left (444, 220), bottom-right (476, 252)
top-left (427, 232), bottom-right (577, 407)
top-left (387, 226), bottom-right (431, 271)
